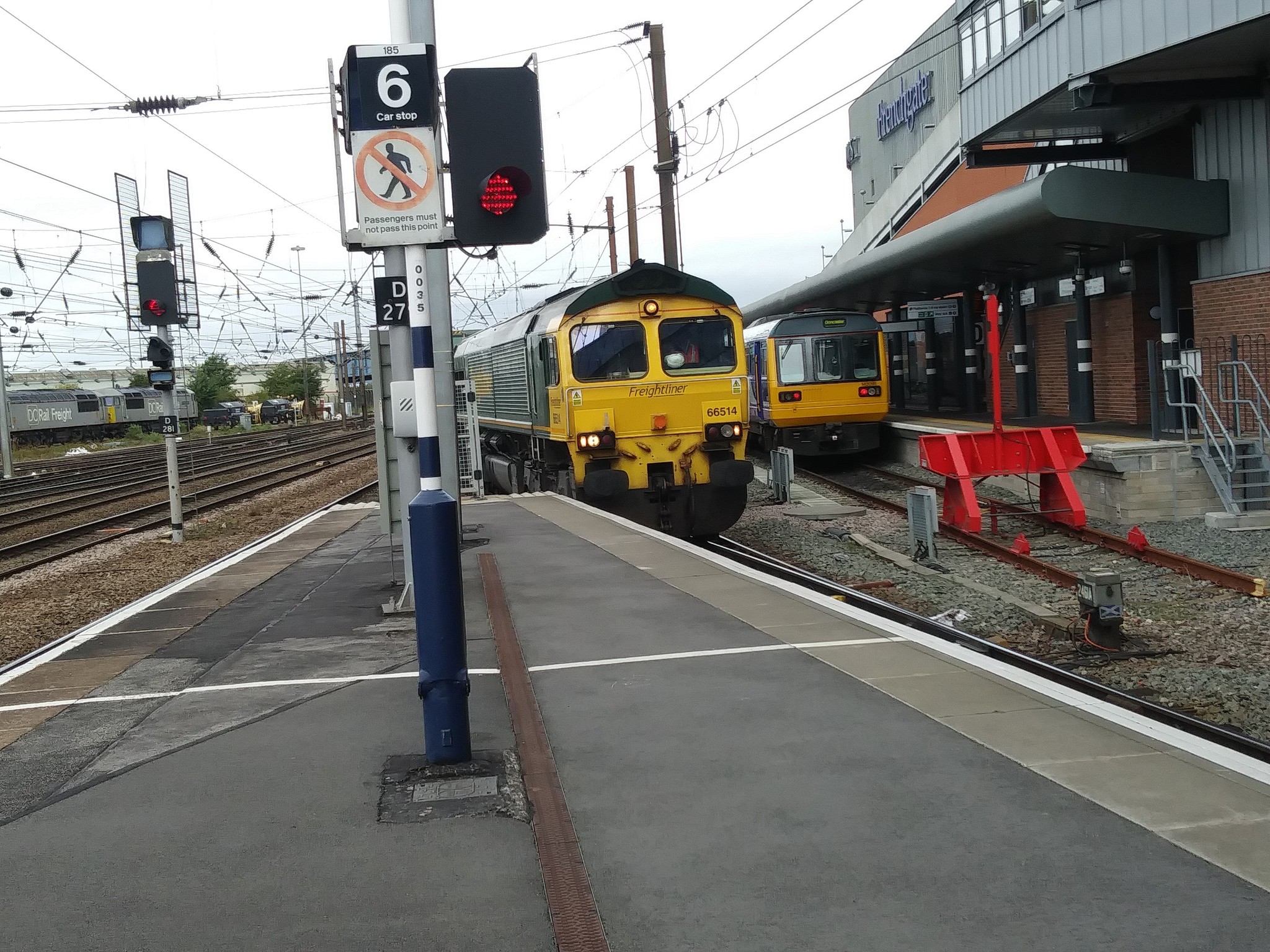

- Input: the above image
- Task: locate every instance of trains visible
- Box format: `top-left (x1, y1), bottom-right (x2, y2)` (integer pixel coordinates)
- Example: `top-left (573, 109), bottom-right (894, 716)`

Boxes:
top-left (727, 308), bottom-right (891, 457)
top-left (4, 388), bottom-right (198, 450)
top-left (451, 259), bottom-right (755, 542)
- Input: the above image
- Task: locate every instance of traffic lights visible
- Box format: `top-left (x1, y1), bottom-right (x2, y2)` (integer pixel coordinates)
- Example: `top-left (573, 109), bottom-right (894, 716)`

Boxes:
top-left (444, 66), bottom-right (549, 246)
top-left (147, 336), bottom-right (173, 362)
top-left (136, 261), bottom-right (178, 325)
top-left (129, 217), bottom-right (174, 250)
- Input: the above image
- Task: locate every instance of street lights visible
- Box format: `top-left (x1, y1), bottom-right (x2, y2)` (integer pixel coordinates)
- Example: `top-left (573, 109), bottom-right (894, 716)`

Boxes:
top-left (291, 246), bottom-right (311, 423)
